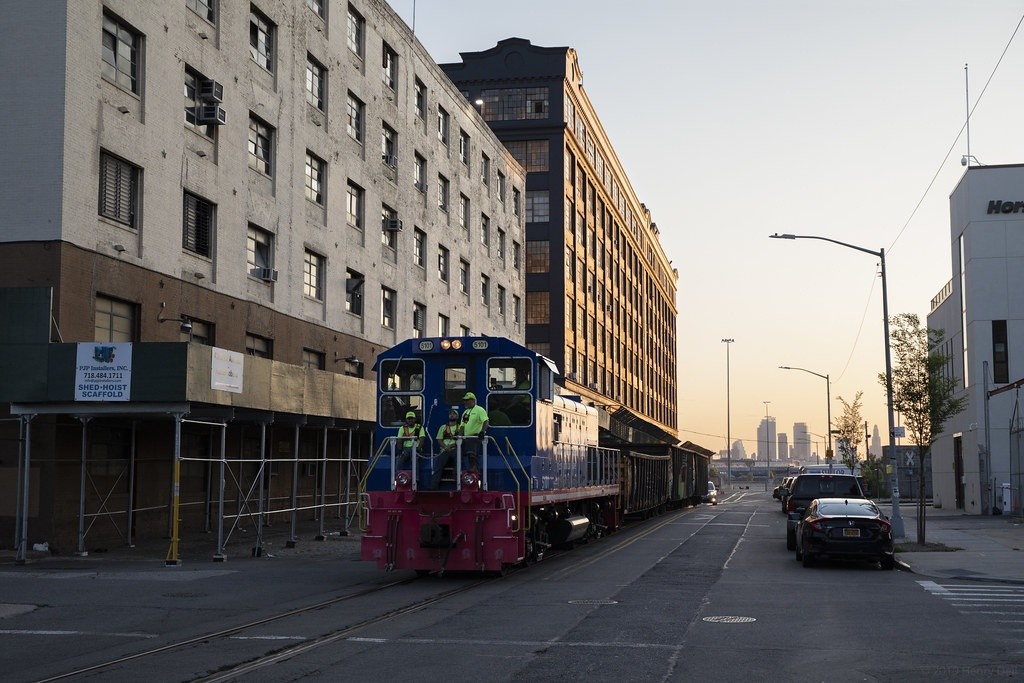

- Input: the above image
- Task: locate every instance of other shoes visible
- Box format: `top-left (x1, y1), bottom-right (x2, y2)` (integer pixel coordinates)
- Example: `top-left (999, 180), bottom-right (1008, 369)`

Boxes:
top-left (419, 511), bottom-right (430, 516)
top-left (441, 511), bottom-right (451, 516)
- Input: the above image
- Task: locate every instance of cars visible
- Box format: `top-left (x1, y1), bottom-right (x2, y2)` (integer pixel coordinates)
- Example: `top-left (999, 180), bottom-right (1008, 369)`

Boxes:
top-left (771, 474), bottom-right (896, 571)
top-left (703, 481), bottom-right (718, 505)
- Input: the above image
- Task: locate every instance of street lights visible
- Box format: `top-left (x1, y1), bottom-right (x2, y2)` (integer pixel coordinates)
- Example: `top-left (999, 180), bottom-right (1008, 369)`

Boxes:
top-left (721, 338), bottom-right (736, 485)
top-left (778, 366), bottom-right (832, 473)
top-left (763, 401), bottom-right (772, 478)
top-left (801, 431), bottom-right (827, 463)
top-left (768, 232), bottom-right (906, 538)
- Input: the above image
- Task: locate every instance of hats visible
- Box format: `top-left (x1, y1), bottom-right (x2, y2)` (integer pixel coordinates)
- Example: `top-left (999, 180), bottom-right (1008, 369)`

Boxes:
top-left (448, 410), bottom-right (459, 419)
top-left (406, 411), bottom-right (415, 418)
top-left (462, 392), bottom-right (476, 399)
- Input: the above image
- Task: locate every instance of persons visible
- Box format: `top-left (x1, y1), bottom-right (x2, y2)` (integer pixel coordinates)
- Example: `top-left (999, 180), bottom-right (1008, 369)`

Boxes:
top-left (505, 370), bottom-right (530, 426)
top-left (430, 409), bottom-right (460, 491)
top-left (458, 392), bottom-right (489, 474)
top-left (395, 411), bottom-right (425, 487)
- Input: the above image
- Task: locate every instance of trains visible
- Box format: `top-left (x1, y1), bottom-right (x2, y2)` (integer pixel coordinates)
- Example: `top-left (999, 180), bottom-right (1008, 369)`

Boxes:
top-left (358, 335), bottom-right (715, 571)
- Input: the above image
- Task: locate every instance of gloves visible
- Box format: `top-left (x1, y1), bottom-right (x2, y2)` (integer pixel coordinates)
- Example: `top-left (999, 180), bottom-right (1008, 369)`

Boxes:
top-left (479, 431), bottom-right (484, 440)
